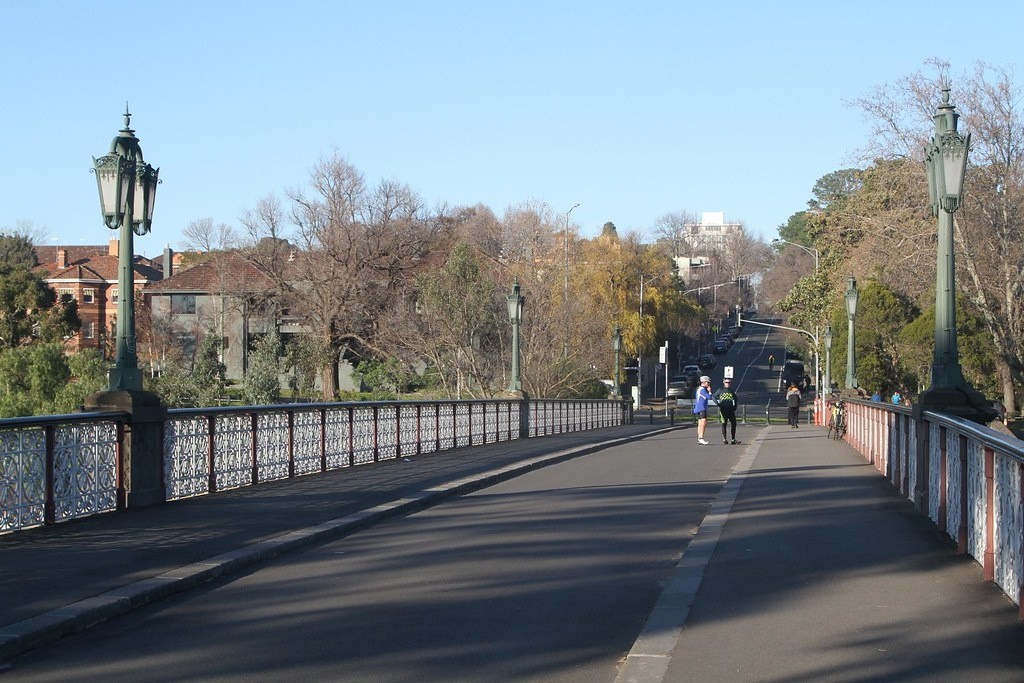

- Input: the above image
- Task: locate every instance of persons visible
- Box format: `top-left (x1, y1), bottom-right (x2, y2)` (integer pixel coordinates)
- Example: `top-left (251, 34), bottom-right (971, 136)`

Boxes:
top-left (712, 379), bottom-right (741, 445)
top-left (787, 374), bottom-right (811, 429)
top-left (870, 390), bottom-right (911, 407)
top-left (693, 376), bottom-right (712, 445)
top-left (768, 355), bottom-right (775, 370)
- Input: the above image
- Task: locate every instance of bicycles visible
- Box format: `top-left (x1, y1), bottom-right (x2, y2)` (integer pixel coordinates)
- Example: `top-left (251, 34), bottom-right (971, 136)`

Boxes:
top-left (825, 399), bottom-right (848, 442)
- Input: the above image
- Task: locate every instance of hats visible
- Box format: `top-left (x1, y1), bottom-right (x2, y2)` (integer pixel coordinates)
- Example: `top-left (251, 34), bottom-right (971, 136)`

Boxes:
top-left (700, 376), bottom-right (711, 382)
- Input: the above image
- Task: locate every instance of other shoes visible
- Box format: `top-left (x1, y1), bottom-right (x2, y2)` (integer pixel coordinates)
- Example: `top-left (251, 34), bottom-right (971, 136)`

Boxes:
top-left (731, 439), bottom-right (741, 444)
top-left (721, 439), bottom-right (728, 444)
top-left (697, 439), bottom-right (708, 445)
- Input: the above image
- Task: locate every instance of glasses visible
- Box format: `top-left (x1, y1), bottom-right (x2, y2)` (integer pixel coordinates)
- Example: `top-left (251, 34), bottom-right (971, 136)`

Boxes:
top-left (725, 382), bottom-right (731, 384)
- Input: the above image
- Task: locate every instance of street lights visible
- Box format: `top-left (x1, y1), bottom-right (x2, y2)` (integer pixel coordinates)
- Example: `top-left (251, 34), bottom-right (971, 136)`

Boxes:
top-left (504, 273), bottom-right (526, 394)
top-left (611, 324), bottom-right (626, 399)
top-left (772, 239), bottom-right (821, 402)
top-left (823, 322), bottom-right (832, 392)
top-left (842, 270), bottom-right (858, 391)
top-left (918, 85), bottom-right (986, 404)
top-left (90, 101), bottom-right (161, 383)
top-left (637, 263), bottom-right (712, 408)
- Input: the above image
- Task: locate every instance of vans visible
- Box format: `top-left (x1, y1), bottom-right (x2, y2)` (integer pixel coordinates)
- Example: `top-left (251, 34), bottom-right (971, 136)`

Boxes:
top-left (779, 360), bottom-right (804, 393)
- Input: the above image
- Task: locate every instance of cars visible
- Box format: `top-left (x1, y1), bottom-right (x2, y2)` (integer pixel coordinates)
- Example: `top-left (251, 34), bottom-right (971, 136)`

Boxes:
top-left (695, 324), bottom-right (742, 369)
top-left (666, 381), bottom-right (691, 398)
top-left (670, 375), bottom-right (694, 393)
top-left (681, 365), bottom-right (702, 378)
top-left (685, 371), bottom-right (701, 386)
